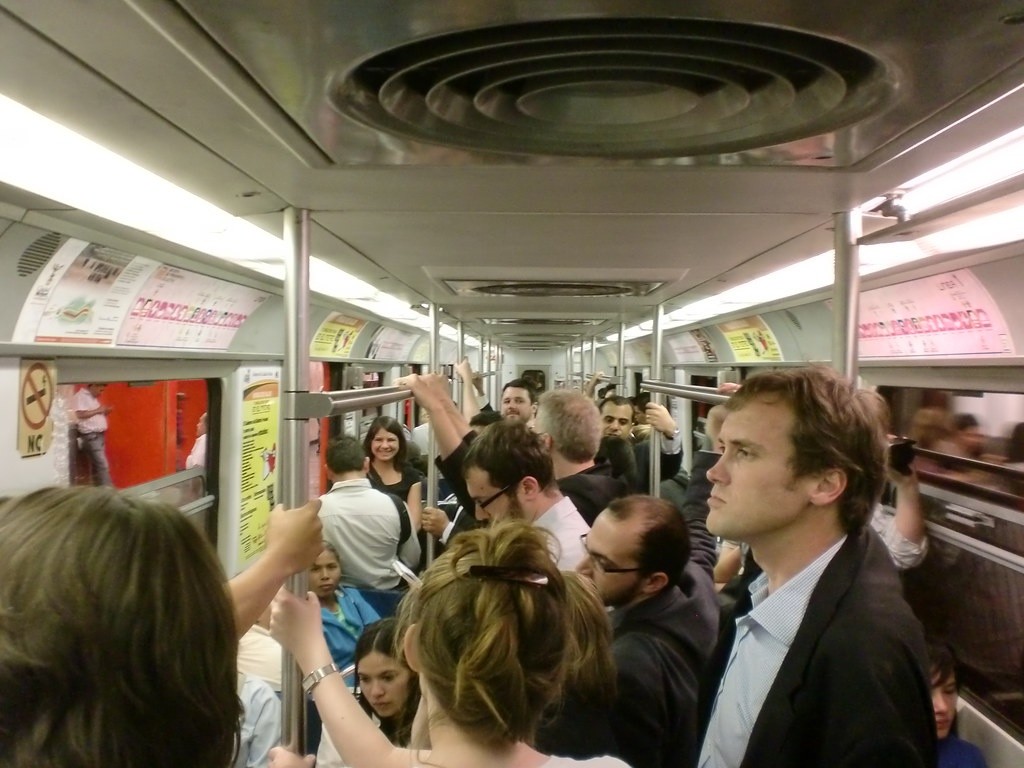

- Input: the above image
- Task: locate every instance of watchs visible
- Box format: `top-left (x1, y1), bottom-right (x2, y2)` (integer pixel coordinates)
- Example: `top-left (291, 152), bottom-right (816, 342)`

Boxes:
top-left (663, 429), bottom-right (680, 440)
top-left (302, 663), bottom-right (339, 694)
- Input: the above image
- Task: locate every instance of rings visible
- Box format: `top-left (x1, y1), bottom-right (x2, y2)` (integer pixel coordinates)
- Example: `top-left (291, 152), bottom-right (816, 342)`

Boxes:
top-left (647, 414), bottom-right (650, 419)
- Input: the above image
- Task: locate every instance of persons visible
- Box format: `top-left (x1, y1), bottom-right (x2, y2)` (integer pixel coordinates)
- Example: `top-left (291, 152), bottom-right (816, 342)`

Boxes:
top-left (318, 618), bottom-right (431, 768)
top-left (73, 382), bottom-right (113, 488)
top-left (924, 642), bottom-right (986, 767)
top-left (873, 434), bottom-right (929, 567)
top-left (884, 407), bottom-right (1024, 725)
top-left (0, 485), bottom-right (325, 768)
top-left (538, 377), bottom-right (761, 768)
top-left (185, 411), bottom-right (207, 498)
top-left (308, 540), bottom-right (379, 686)
top-left (270, 520), bottom-right (631, 768)
top-left (314, 358), bottom-right (745, 594)
top-left (691, 365), bottom-right (938, 768)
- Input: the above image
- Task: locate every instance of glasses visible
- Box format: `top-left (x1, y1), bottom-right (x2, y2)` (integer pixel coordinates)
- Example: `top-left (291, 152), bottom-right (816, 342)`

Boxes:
top-left (474, 477), bottom-right (524, 511)
top-left (580, 533), bottom-right (651, 573)
